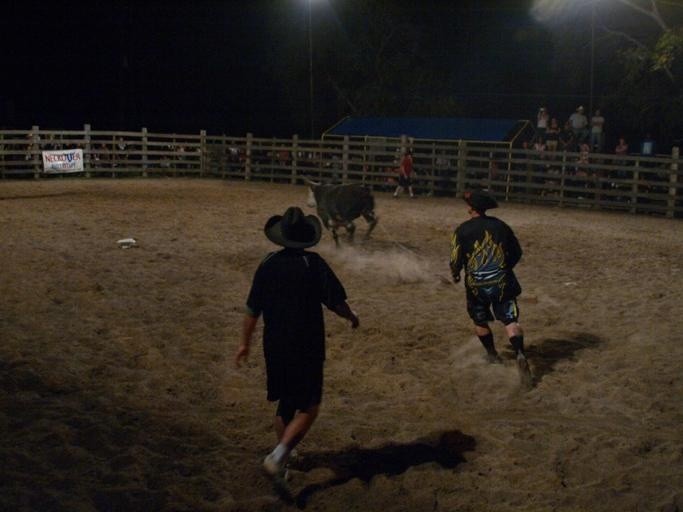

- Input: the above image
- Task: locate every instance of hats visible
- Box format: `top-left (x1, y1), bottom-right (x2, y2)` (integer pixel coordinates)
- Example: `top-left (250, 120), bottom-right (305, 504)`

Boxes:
top-left (463, 190), bottom-right (497, 209)
top-left (264, 207), bottom-right (322, 249)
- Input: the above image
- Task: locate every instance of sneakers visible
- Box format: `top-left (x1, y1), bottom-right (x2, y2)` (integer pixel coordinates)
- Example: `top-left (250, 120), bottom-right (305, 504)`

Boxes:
top-left (258, 454), bottom-right (297, 495)
top-left (517, 353), bottom-right (533, 392)
top-left (284, 453), bottom-right (312, 471)
top-left (482, 354), bottom-right (503, 364)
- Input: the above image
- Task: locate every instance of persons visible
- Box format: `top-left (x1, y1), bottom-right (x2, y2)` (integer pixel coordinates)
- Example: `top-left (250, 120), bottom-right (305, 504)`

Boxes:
top-left (234, 206), bottom-right (360, 493)
top-left (392, 145), bottom-right (417, 199)
top-left (523, 104), bottom-right (632, 199)
top-left (448, 189), bottom-right (533, 389)
top-left (640, 130), bottom-right (657, 177)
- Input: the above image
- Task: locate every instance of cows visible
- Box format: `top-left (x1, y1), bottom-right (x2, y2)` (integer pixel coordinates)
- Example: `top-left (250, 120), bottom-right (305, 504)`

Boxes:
top-left (300, 175), bottom-right (379, 249)
